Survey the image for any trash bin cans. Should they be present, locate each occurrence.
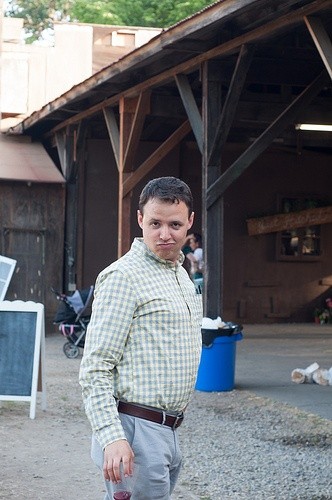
[196,322,243,391]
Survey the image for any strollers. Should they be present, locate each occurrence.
[49,283,95,359]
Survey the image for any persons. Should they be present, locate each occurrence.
[184,234,203,274]
[78,175,203,500]
[179,244,198,278]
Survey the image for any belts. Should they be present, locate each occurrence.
[116,399,184,430]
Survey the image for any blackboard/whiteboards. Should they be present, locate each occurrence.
[0,301,46,401]
